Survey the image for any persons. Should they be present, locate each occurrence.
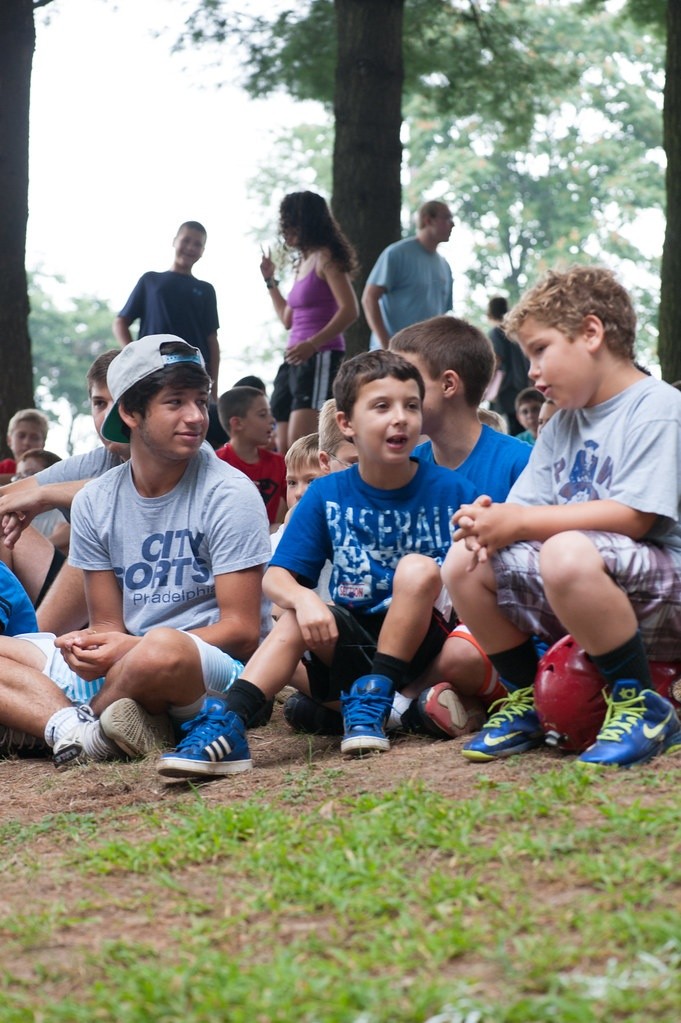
[277,315,552,739]
[0,333,272,771]
[362,199,454,350]
[482,296,537,436]
[0,346,680,724]
[440,266,681,767]
[259,190,361,455]
[156,348,481,774]
[112,220,221,405]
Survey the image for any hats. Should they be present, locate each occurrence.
[100,334,206,444]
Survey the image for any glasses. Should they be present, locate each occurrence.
[11,472,35,482]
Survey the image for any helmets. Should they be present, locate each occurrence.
[535,635,676,754]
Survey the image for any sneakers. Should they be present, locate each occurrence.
[52,697,175,774]
[461,677,544,763]
[282,692,345,736]
[339,674,401,753]
[401,682,486,739]
[574,680,681,772]
[157,697,253,778]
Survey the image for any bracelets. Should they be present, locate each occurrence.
[266,275,281,289]
[307,337,321,352]
